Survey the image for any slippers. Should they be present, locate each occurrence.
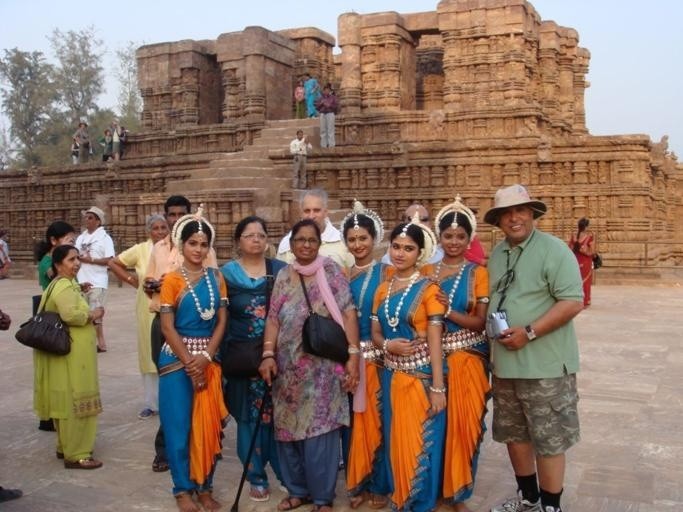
[248,484,270,502]
[96,345,107,352]
[310,504,334,512]
[277,495,312,512]
[369,494,387,509]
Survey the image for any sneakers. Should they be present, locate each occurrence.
[489,489,542,512]
[137,408,154,420]
[151,456,170,472]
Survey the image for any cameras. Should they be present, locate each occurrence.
[486,312,511,338]
[149,280,161,293]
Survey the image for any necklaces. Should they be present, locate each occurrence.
[345,259,376,318]
[178,261,220,322]
[433,256,467,321]
[439,257,469,269]
[181,264,204,276]
[383,266,421,333]
[353,261,374,270]
[390,271,415,283]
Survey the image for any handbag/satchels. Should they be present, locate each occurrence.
[222,339,263,380]
[14,275,73,356]
[301,311,350,365]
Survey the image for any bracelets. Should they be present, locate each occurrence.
[199,349,213,363]
[427,383,445,394]
[523,322,537,343]
[88,255,96,264]
[124,271,135,285]
[258,338,278,366]
[380,336,392,360]
[346,340,359,364]
[443,303,452,320]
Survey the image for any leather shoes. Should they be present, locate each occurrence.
[55,450,95,459]
[63,458,104,470]
[0,485,23,501]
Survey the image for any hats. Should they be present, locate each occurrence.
[483,183,549,227]
[80,206,106,226]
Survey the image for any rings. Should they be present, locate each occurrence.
[193,366,206,390]
[341,369,359,392]
[432,405,441,412]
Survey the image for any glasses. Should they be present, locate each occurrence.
[293,236,320,246]
[240,232,266,241]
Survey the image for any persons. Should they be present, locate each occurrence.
[422,212,489,511]
[220,215,290,502]
[33,219,81,289]
[339,213,394,511]
[258,219,360,511]
[157,221,228,512]
[143,196,215,473]
[106,215,164,420]
[98,130,111,160]
[289,129,311,189]
[72,206,113,355]
[0,309,22,504]
[314,83,338,148]
[119,126,126,159]
[74,119,91,162]
[302,70,318,119]
[274,189,352,266]
[486,187,585,511]
[292,80,303,118]
[37,245,103,469]
[109,117,124,162]
[0,229,11,277]
[568,219,595,306]
[371,221,445,512]
[70,137,80,163]
